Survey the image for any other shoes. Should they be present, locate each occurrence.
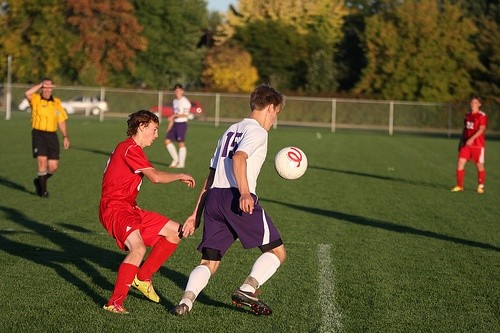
[476,187,484,193]
[34,178,48,198]
[450,185,465,192]
[169,160,185,169]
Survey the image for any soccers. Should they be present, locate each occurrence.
[274,146,308,180]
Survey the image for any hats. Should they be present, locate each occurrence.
[174,84,182,89]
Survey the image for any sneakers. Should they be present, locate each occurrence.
[173,302,189,316]
[231,286,273,316]
[131,273,161,303]
[102,304,130,314]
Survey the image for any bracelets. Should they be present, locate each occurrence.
[63,136,68,138]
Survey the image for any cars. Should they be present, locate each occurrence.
[148,98,204,121]
[18,96,75,115]
[66,95,110,117]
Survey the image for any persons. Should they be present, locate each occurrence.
[173,85,286,316]
[24,78,70,198]
[99,109,196,315]
[165,83,192,169]
[450,96,487,194]
